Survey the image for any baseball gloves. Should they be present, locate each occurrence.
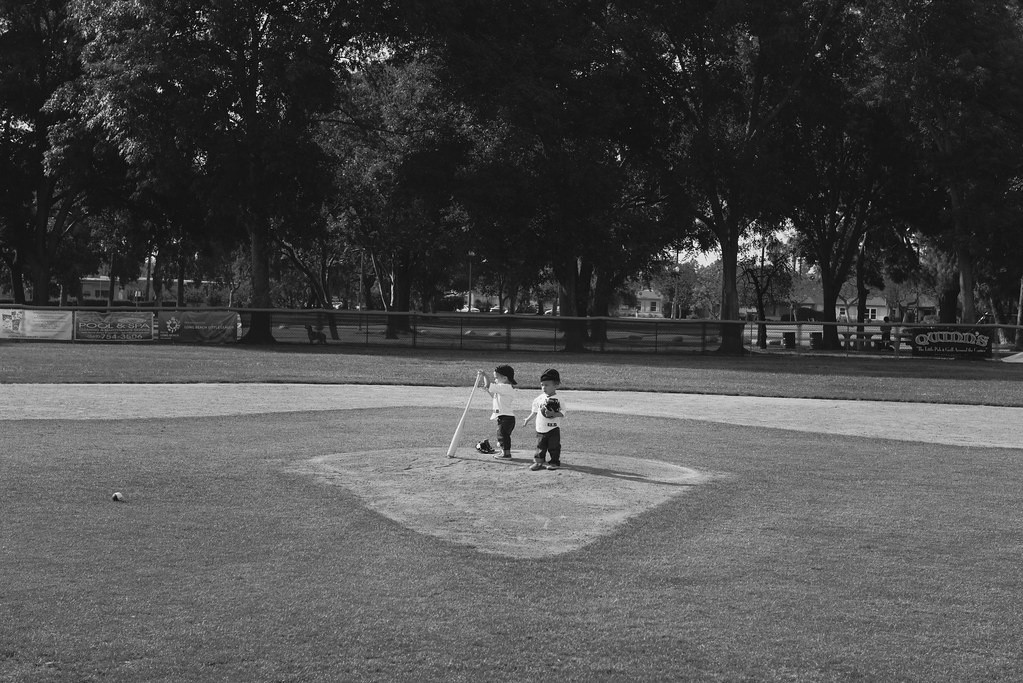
[541,398,561,418]
[475,439,495,454]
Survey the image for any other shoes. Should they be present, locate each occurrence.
[530,462,543,470]
[494,453,511,460]
[547,463,559,470]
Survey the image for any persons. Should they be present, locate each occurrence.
[477,364,517,459]
[522,368,565,471]
[881,318,891,340]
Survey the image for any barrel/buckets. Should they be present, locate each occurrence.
[809,332,822,349]
[782,332,796,351]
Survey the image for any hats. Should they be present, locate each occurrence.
[540,369,562,385]
[494,365,517,385]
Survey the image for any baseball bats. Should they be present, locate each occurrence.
[447,369,483,457]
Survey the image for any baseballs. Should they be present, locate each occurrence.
[112,492,123,501]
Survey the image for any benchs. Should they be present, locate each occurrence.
[840,339,872,349]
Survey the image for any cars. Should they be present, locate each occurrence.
[457,305,481,312]
[490,305,510,313]
[545,305,560,316]
